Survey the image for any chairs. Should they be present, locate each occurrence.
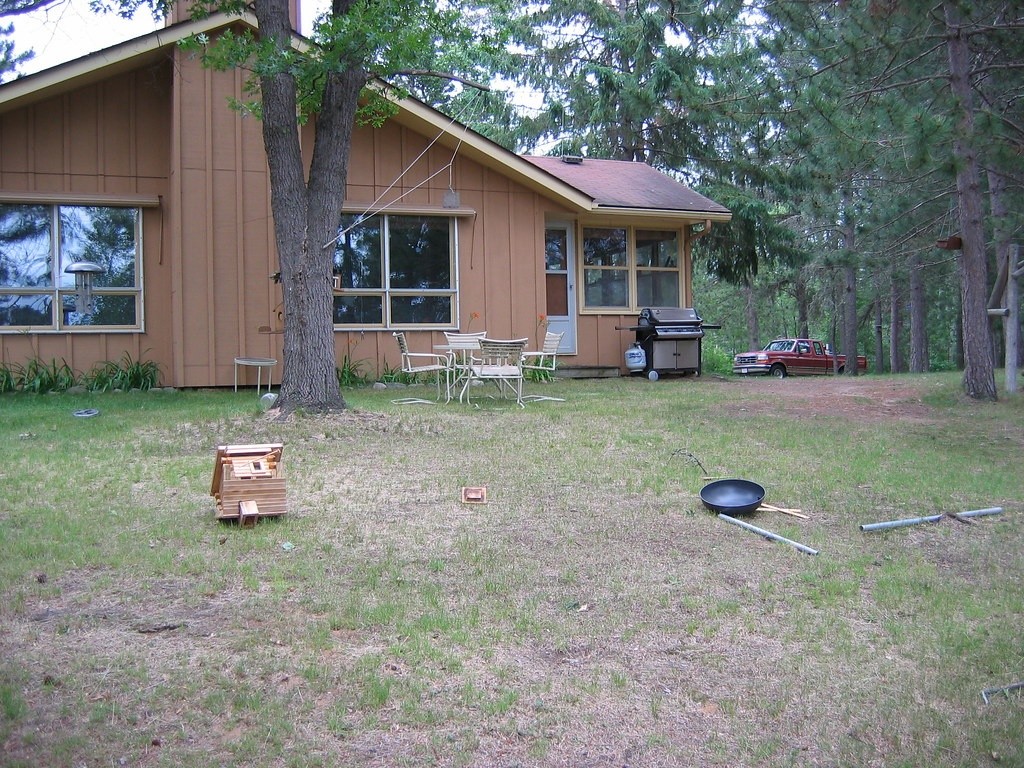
[391,330,566,411]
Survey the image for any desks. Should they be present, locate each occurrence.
[433,343,529,404]
[235,357,281,396]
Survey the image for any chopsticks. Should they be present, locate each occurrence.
[754,503,810,520]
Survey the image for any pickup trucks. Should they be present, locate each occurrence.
[731,338,867,379]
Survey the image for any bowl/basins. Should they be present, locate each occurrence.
[699,478,766,515]
